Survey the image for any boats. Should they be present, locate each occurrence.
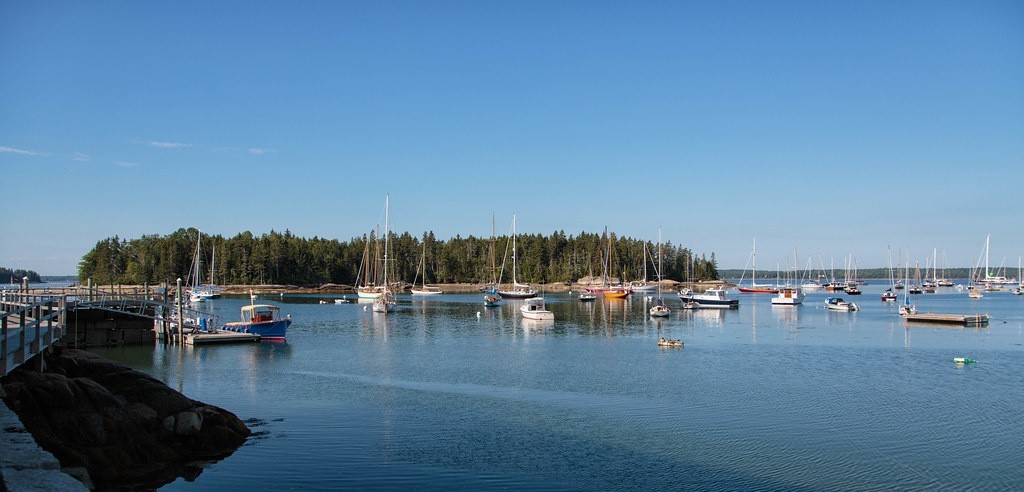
[678,286,740,309]
[173,290,192,310]
[520,276,554,319]
[683,302,699,309]
[657,339,685,346]
[772,287,806,305]
[824,296,852,310]
[222,288,292,343]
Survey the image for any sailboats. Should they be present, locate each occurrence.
[410,242,445,296]
[478,243,502,294]
[497,212,539,299]
[778,233,1024,302]
[484,212,505,307]
[352,223,414,302]
[649,227,671,317]
[577,231,683,301]
[181,229,207,303]
[194,245,222,299]
[372,193,398,314]
[897,250,918,315]
[736,238,779,293]
